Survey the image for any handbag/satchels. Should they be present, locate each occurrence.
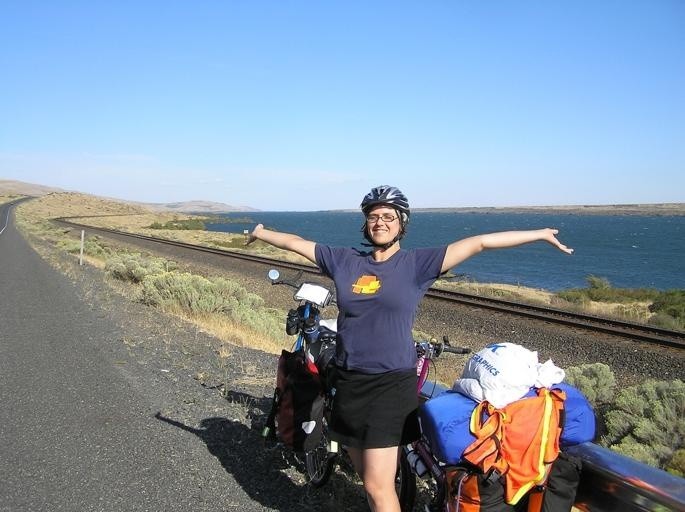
[453,340,567,414]
[275,346,325,454]
[442,449,581,512]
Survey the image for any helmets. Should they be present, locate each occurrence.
[362,184,411,217]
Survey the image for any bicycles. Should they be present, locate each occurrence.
[394,333,474,512]
[266,271,345,488]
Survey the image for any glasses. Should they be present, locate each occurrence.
[363,212,399,223]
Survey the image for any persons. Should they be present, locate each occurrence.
[244,186,575,512]
